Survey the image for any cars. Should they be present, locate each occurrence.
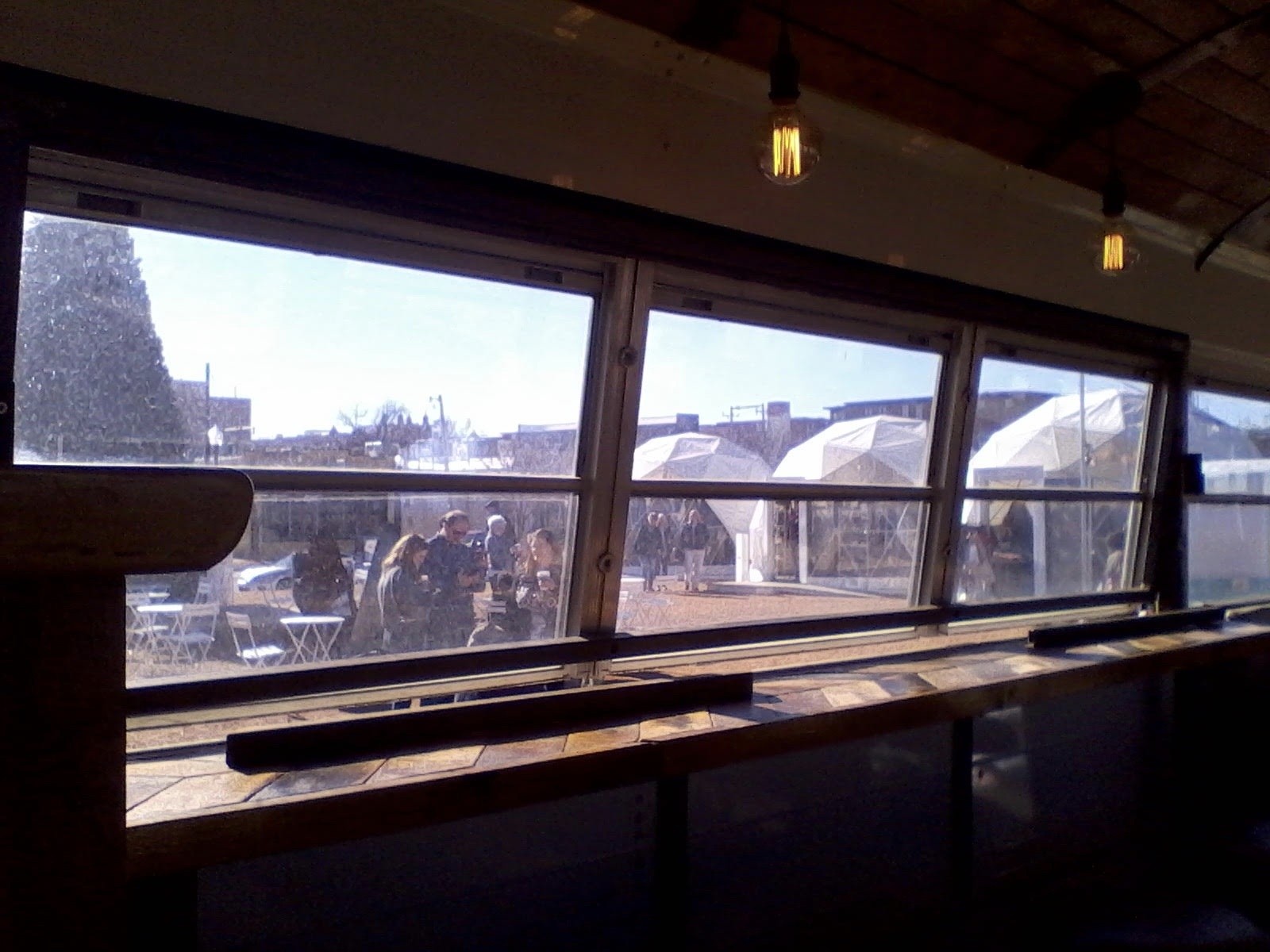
[237,552,355,591]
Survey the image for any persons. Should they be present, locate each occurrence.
[292,498,1127,712]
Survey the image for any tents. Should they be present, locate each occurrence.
[631,388,1269,620]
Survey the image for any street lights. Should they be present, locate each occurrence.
[206,424,224,465]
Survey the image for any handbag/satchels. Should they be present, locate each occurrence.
[972,558,996,585]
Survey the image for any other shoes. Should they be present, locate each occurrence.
[648,586,654,591]
[685,584,689,590]
[692,588,699,593]
[643,581,647,591]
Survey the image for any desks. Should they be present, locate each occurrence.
[279,616,345,662]
[135,604,186,664]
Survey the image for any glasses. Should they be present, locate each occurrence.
[448,525,466,537]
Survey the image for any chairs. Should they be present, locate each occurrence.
[123,583,286,669]
